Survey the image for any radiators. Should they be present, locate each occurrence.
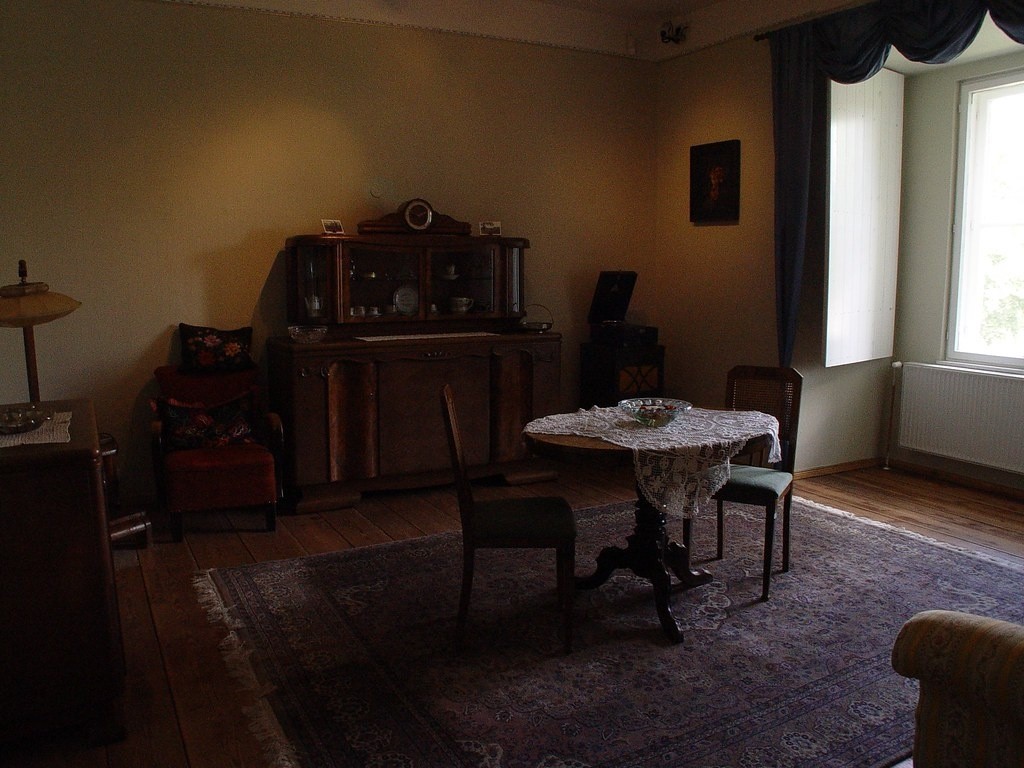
[882,361,1024,476]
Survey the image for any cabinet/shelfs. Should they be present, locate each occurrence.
[0,398,127,748]
[581,342,664,408]
[266,234,564,518]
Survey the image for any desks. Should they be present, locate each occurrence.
[526,408,770,643]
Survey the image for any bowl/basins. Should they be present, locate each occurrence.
[618,398,692,426]
[287,325,328,342]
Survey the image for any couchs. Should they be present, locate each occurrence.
[890,610,1024,768]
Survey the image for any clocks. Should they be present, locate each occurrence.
[357,198,472,236]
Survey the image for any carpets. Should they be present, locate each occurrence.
[191,499,1024,768]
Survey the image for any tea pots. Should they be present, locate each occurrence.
[305,294,324,316]
[450,298,474,311]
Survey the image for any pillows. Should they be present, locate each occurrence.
[178,322,260,373]
[148,386,260,452]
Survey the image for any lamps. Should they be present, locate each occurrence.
[0,258,82,402]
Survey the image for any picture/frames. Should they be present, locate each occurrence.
[689,139,740,227]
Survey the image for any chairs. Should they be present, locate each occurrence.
[440,381,578,655]
[146,366,288,542]
[683,365,805,602]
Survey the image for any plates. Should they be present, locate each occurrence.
[353,314,366,317]
[393,285,421,316]
[366,314,382,317]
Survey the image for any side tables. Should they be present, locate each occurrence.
[97,433,151,545]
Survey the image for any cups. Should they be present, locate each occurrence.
[354,305,365,314]
[369,307,380,314]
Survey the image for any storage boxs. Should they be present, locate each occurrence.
[588,271,659,341]
